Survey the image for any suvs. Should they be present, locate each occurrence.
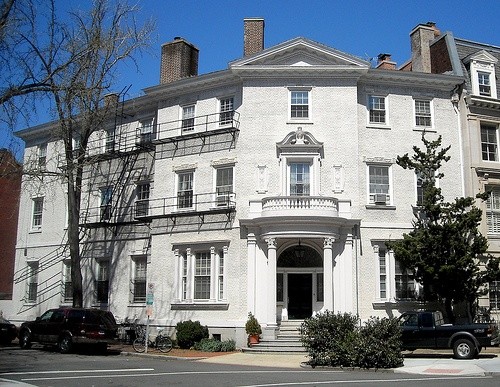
[17,308,120,356]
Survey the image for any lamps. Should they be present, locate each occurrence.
[294,238,305,259]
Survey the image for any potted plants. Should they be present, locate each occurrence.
[245,311,262,344]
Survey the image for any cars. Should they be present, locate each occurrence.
[0,316,18,344]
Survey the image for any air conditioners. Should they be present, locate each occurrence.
[217,196,229,205]
[374,194,386,202]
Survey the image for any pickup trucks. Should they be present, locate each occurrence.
[385,311,497,360]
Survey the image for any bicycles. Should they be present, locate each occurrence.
[132,327,173,353]
